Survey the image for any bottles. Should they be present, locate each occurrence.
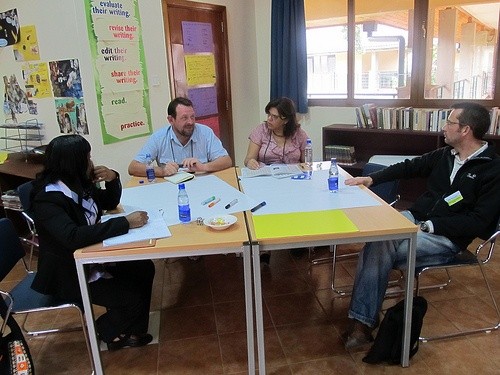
[177,183,191,223]
[304,139,312,168]
[145,154,156,183]
[329,158,338,192]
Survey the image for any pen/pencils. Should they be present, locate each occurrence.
[179,163,196,168]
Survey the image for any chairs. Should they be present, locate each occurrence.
[384,212,500,343]
[306,162,405,297]
[0,217,97,375]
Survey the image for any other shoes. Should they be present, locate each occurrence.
[341,313,380,350]
[259,251,270,265]
[95,322,152,351]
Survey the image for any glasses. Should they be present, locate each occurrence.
[266,112,281,120]
[445,118,466,126]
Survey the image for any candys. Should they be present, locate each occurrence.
[210,218,229,226]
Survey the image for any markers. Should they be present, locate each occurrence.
[251,202,266,212]
[225,199,237,209]
[202,196,215,205]
[209,198,221,207]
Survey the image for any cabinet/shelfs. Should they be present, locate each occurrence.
[322,124,500,210]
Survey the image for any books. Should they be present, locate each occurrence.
[359,104,453,132]
[164,171,195,184]
[325,145,356,166]
[485,107,500,135]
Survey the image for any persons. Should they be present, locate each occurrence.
[245,97,308,264]
[128,97,233,176]
[342,101,500,349]
[30,134,155,349]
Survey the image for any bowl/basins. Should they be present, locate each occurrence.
[203,215,238,230]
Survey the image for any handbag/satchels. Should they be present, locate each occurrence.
[0,332,34,375]
[361,296,428,365]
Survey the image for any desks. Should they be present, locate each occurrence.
[0,151,44,235]
[73,166,256,375]
[235,161,419,375]
